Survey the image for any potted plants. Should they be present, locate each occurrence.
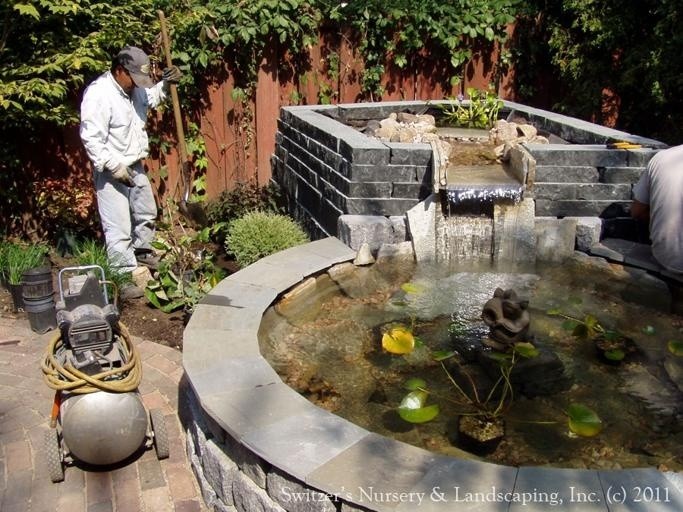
[7,244,46,312]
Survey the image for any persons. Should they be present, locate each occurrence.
[79,45,182,301]
[630,143,683,274]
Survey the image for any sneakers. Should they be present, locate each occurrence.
[118,281,143,300]
[134,250,160,269]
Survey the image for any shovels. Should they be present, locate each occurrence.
[156,9,209,231]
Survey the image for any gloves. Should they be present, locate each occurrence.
[162,65,180,82]
[111,162,136,187]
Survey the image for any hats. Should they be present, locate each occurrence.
[117,46,153,88]
